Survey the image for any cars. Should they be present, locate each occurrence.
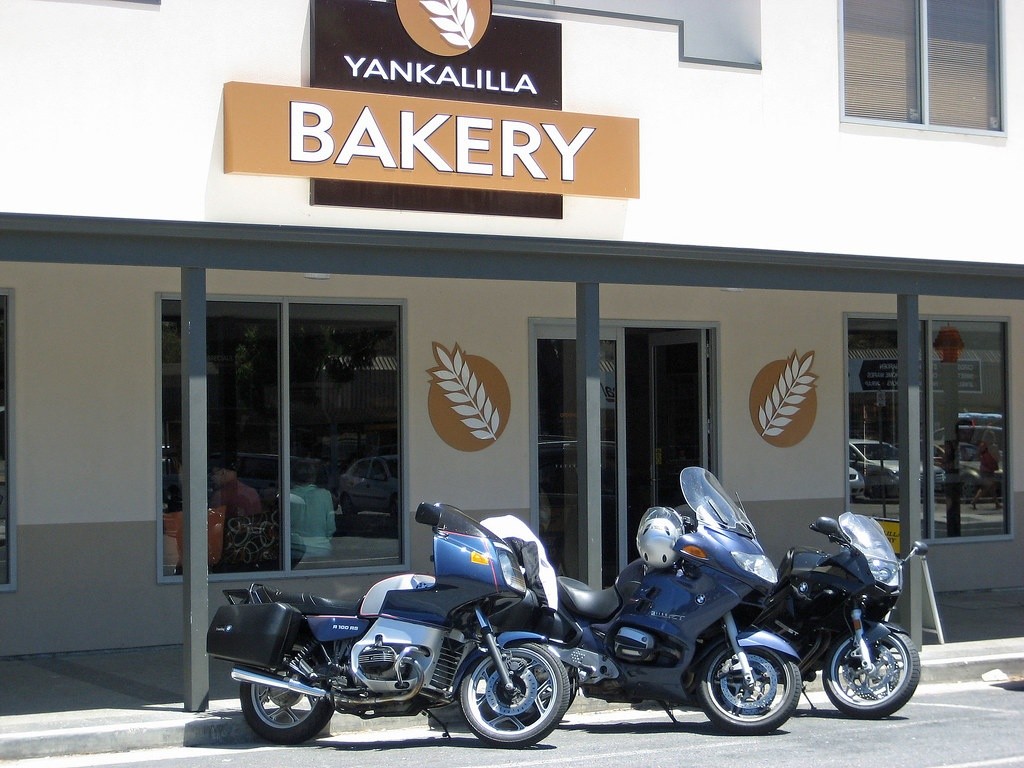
[848,464,864,503]
[161,455,182,513]
[335,452,399,523]
[848,435,947,500]
[889,436,1005,498]
[538,433,618,547]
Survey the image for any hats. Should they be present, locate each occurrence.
[212,455,237,470]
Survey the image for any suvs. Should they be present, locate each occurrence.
[211,444,341,512]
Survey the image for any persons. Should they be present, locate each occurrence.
[290,463,337,561]
[972,429,1003,510]
[207,453,262,520]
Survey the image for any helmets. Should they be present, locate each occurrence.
[636,506,684,569]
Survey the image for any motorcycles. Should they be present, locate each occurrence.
[697,511,932,720]
[203,500,573,750]
[477,464,804,735]
